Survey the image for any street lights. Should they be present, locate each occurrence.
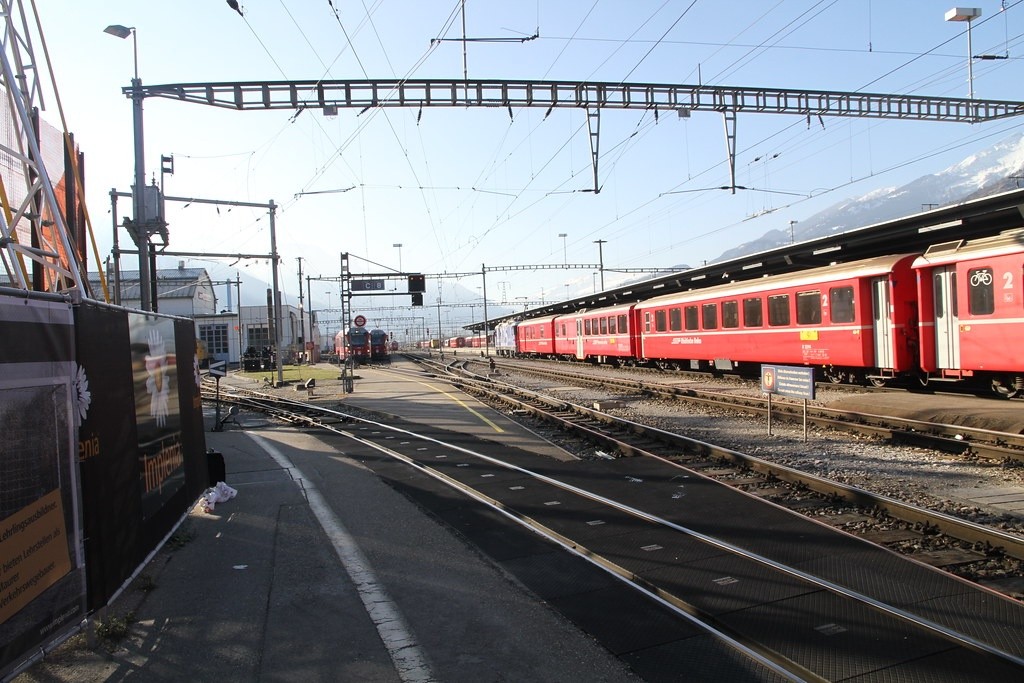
[101,25,168,313]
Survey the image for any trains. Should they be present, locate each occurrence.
[368,329,391,364]
[332,327,371,364]
[494,223,1024,403]
[386,332,495,352]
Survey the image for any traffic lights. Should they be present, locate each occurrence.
[405,329,408,335]
[390,331,392,339]
[426,328,429,335]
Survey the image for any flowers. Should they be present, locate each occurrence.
[50,361,92,429]
[193,353,200,388]
[144,329,171,429]
